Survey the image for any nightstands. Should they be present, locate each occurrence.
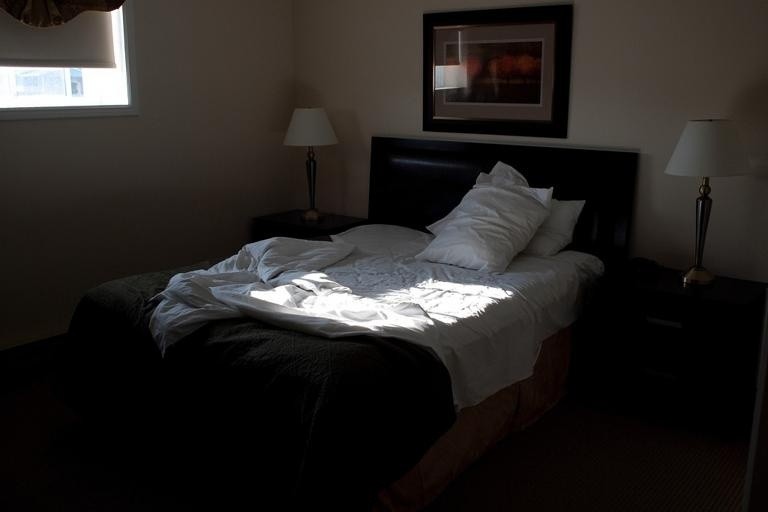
[243,209,365,251]
[619,257,767,445]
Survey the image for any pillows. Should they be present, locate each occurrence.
[414,172,554,275]
[426,186,586,257]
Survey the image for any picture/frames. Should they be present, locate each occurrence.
[422,3,573,139]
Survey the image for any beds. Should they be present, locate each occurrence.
[48,136,638,512]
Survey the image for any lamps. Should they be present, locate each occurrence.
[664,119,750,287]
[282,108,339,221]
